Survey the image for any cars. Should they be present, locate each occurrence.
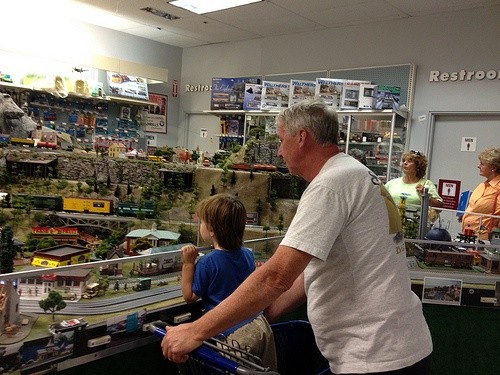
[48,317,89,336]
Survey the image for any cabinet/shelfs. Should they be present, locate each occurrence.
[185,110,405,183]
[0,82,150,159]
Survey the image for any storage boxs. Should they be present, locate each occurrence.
[412,245,476,269]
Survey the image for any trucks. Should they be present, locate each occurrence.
[133,278,152,292]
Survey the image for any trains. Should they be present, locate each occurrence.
[0,192,155,218]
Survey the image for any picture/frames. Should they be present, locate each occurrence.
[144,91,168,134]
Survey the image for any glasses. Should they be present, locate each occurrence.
[410,150,420,155]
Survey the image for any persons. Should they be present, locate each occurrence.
[460,144,500,242]
[384,149,444,252]
[181,193,276,372]
[348,148,368,166]
[161,98,437,375]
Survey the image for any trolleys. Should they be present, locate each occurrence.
[142,319,332,375]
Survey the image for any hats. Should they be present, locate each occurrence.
[426,228,451,241]
